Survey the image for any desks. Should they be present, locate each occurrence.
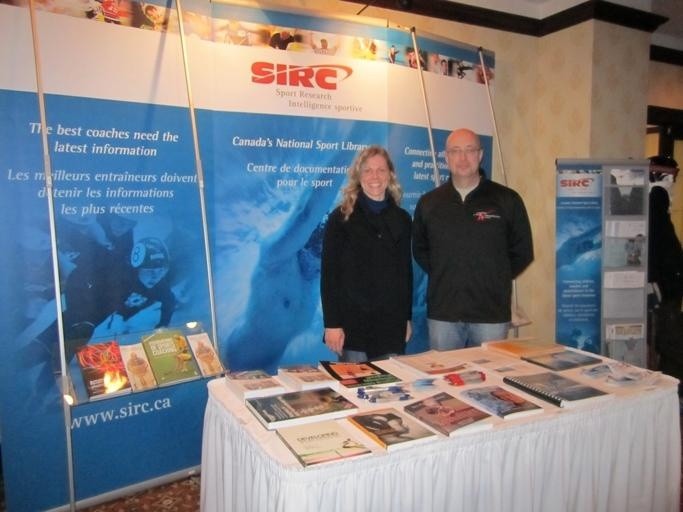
[199,338,682,512]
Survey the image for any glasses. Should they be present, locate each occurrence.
[445,147,480,156]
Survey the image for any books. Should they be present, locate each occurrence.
[221,332,660,464]
[69,329,223,402]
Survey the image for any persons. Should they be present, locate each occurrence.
[322,142,415,365]
[560,327,601,355]
[623,236,644,265]
[83,0,495,86]
[223,119,374,376]
[556,224,604,268]
[411,127,537,351]
